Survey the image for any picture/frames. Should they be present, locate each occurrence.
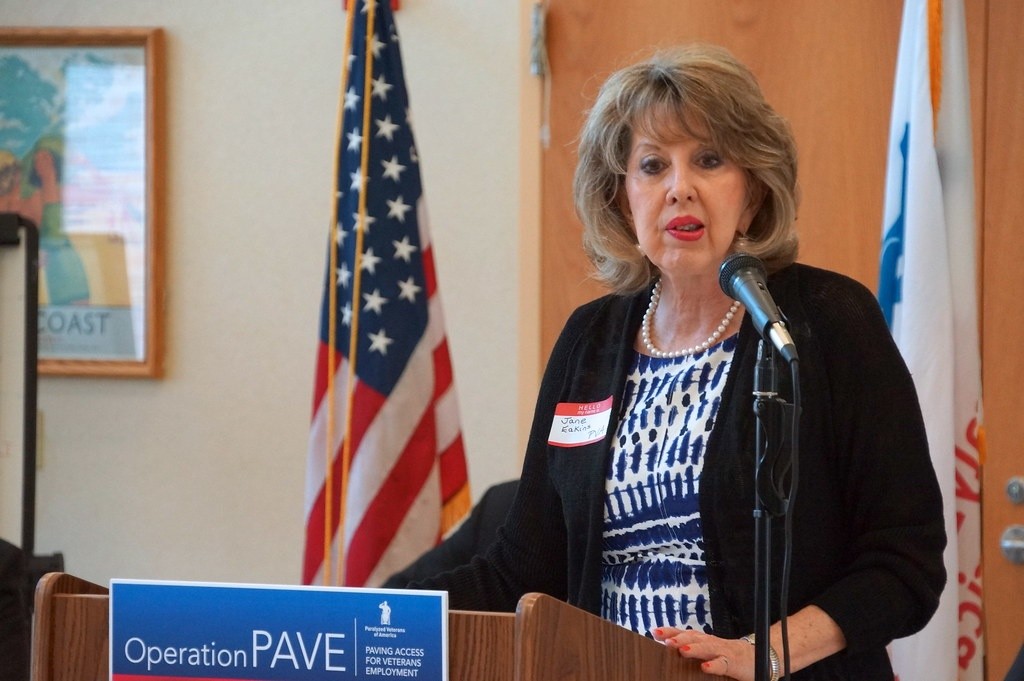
[0,25,168,381]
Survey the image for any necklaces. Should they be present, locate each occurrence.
[642,278,741,358]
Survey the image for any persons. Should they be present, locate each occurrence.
[378,42,949,681]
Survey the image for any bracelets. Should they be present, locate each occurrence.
[740,634,780,681]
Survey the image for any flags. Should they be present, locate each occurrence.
[876,1,983,681]
[300,1,472,588]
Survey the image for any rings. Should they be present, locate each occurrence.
[719,656,729,674]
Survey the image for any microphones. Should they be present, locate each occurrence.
[718,250,800,368]
